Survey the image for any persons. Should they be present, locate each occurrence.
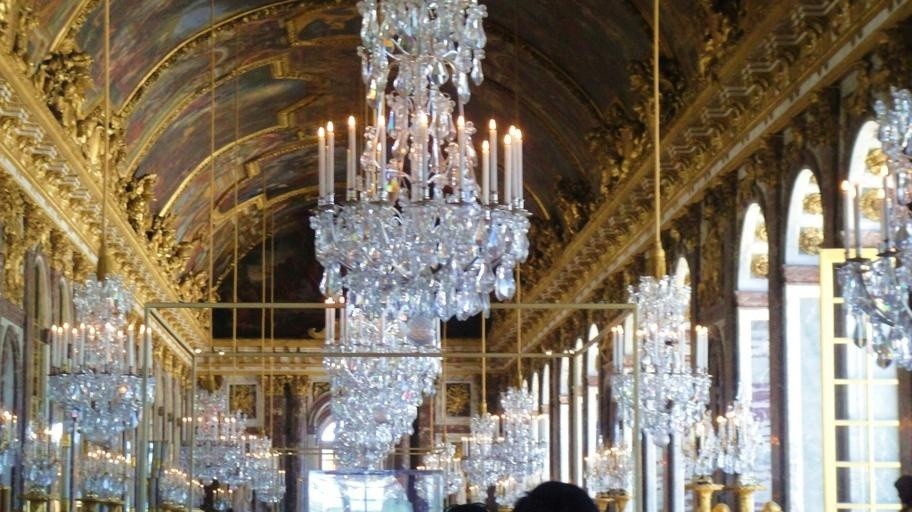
[443,502,491,512]
[512,480,600,512]
[894,474,912,512]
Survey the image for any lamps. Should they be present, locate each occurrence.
[189,2,289,506]
[608,3,715,447]
[44,2,156,445]
[306,1,536,325]
